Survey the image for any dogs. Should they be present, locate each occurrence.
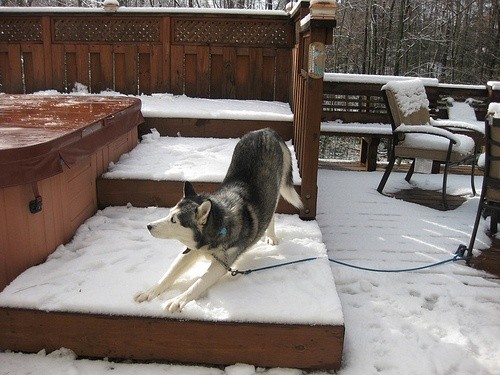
[134,126,305,313]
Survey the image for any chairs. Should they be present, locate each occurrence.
[376,79,484,208]
[466,111,500,265]
